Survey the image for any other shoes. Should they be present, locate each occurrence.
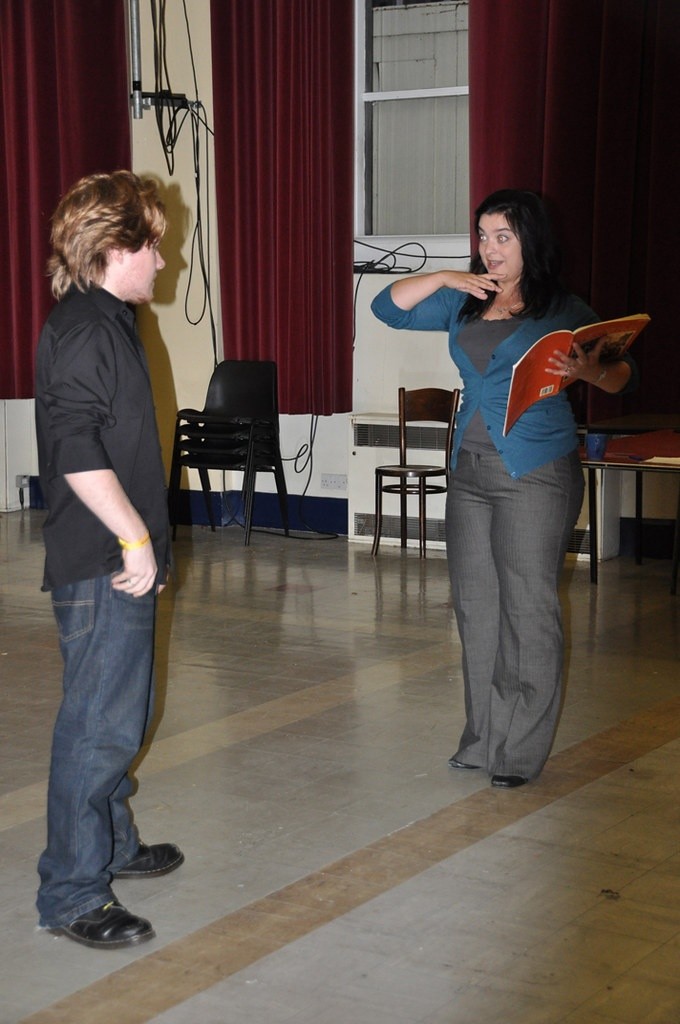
[448,758,481,769]
[491,774,529,788]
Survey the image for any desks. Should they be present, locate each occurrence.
[577,455,680,596]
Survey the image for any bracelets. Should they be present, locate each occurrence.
[115,530,149,549]
[591,365,608,385]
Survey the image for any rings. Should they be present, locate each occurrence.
[128,579,133,586]
[565,366,568,371]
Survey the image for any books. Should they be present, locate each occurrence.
[502,314,650,438]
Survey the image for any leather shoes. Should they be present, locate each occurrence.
[113,840,184,879]
[46,899,155,949]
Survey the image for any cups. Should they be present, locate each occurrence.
[585,433,608,461]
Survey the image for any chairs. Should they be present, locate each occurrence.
[371,387,460,556]
[169,360,289,549]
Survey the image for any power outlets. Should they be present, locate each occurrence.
[320,473,349,489]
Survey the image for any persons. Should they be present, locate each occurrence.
[370,186,637,789]
[32,169,186,951]
